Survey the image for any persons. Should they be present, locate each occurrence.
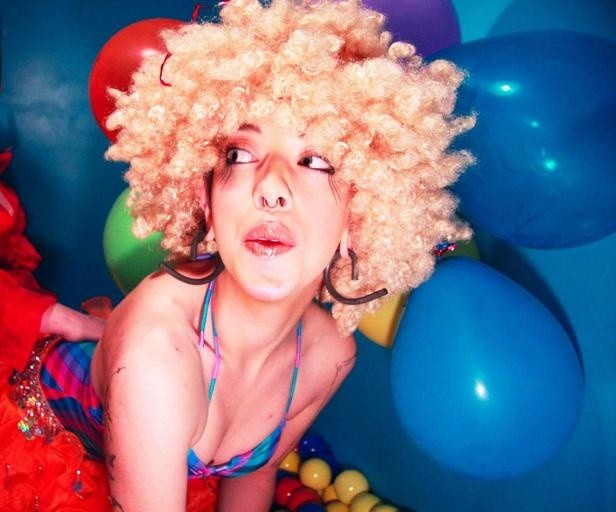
[0,0,479,509]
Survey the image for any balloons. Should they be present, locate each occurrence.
[391,254,582,480]
[358,285,407,349]
[103,183,174,299]
[294,0,462,59]
[419,29,615,249]
[88,16,192,148]
